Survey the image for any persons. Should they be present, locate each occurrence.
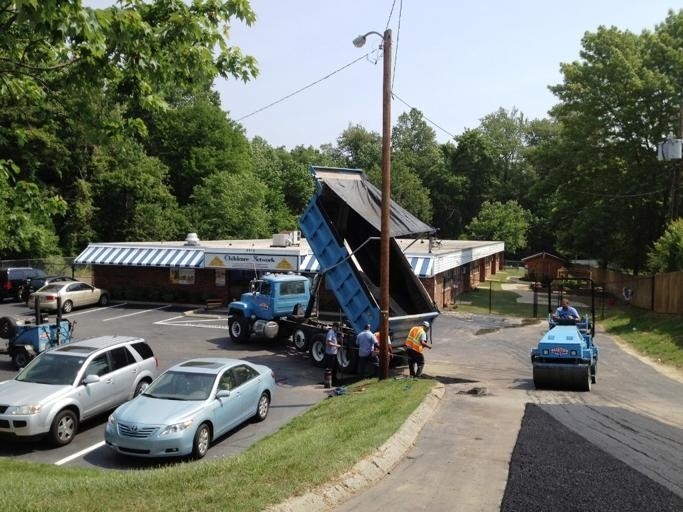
[324,323,341,386]
[355,323,379,379]
[373,326,393,363]
[403,321,431,377]
[552,298,582,322]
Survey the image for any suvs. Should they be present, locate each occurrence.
[0,334,159,448]
[20,275,78,306]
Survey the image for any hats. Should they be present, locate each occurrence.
[424,321,429,327]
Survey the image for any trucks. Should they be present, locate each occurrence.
[226,163,443,379]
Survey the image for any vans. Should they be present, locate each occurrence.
[0,267,47,302]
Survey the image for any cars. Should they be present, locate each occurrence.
[26,280,111,314]
[104,355,277,462]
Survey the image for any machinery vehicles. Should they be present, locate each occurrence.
[0,314,72,366]
[527,312,599,392]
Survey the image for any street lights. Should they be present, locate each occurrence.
[350,28,395,382]
